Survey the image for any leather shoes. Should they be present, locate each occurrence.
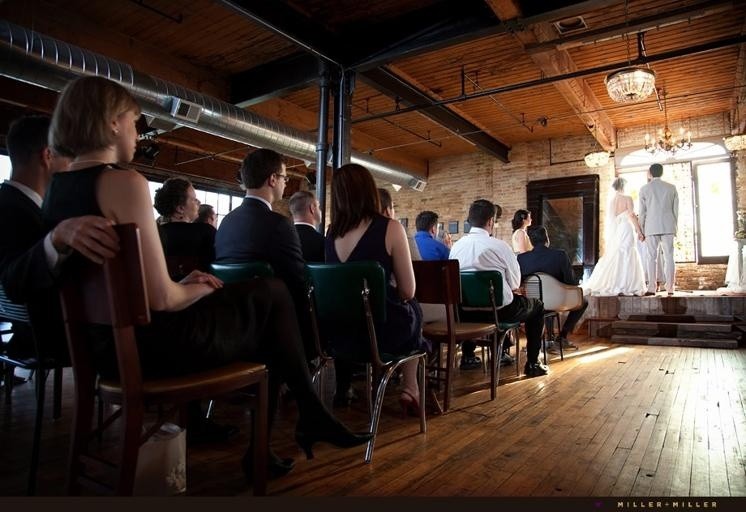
[644,291,656,296]
[524,362,546,377]
[499,353,517,368]
[333,393,362,410]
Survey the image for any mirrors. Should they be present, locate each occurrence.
[526,174,600,284]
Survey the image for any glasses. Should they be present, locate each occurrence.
[276,173,290,182]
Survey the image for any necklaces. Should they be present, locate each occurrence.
[68,160,108,166]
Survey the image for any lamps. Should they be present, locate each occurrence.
[643,93,694,154]
[584,119,609,169]
[602,10,656,105]
[721,108,746,154]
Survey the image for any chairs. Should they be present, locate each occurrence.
[62,223,268,502]
[0,293,105,500]
[526,270,584,356]
[305,264,428,461]
[519,273,564,365]
[408,259,500,412]
[454,269,522,386]
[207,262,324,456]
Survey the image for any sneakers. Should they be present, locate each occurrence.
[547,334,576,352]
[460,356,483,370]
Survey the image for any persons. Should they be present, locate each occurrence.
[578,178,647,297]
[639,170,667,292]
[39,75,374,480]
[153,148,588,418]
[638,164,679,296]
[0,115,240,445]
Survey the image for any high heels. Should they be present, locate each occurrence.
[241,457,296,487]
[398,390,427,420]
[293,421,375,461]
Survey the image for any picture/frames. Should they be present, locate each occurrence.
[463,220,472,232]
[438,222,444,239]
[448,221,458,234]
[399,217,408,228]
